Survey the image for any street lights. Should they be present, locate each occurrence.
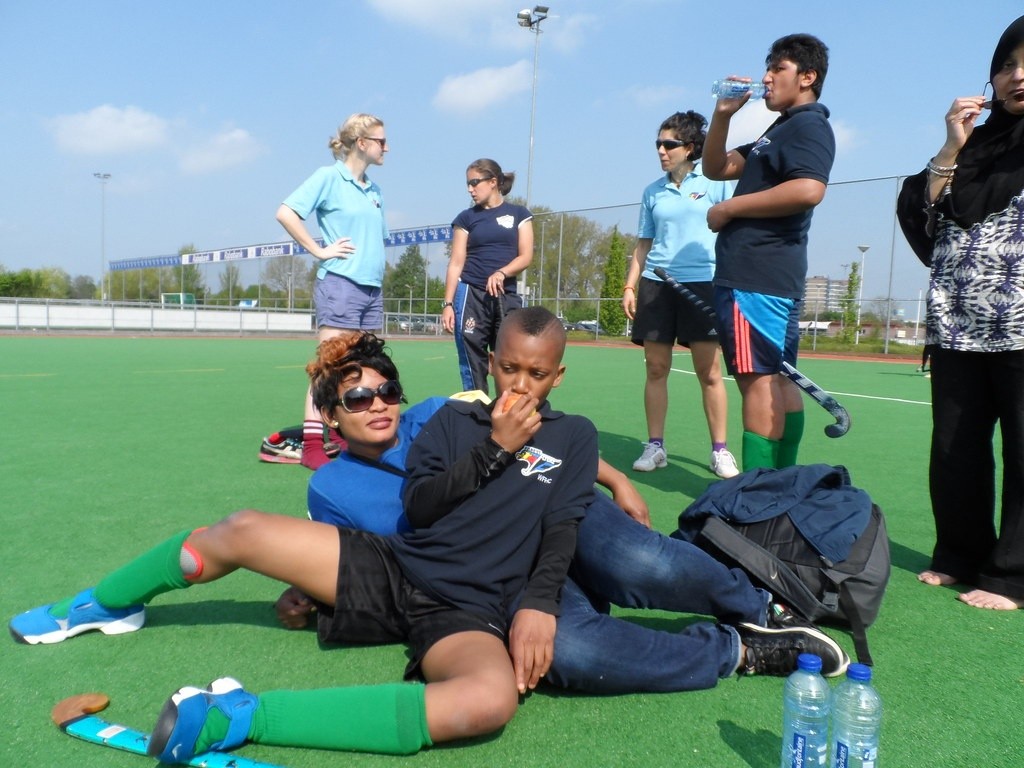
[539,219,551,306]
[405,284,412,335]
[93,172,110,307]
[517,4,550,304]
[856,245,871,344]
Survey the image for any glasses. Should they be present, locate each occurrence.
[981,81,1024,110]
[467,177,492,187]
[356,137,386,148]
[336,381,400,412]
[655,139,686,150]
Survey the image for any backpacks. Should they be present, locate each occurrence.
[668,501,891,627]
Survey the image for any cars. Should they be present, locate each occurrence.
[559,317,606,335]
[386,316,435,330]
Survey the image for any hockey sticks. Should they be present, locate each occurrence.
[497,289,505,319]
[52,693,288,768]
[653,265,850,438]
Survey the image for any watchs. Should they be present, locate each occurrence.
[441,301,452,308]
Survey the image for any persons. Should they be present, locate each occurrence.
[276,334,849,693]
[898,14,1024,611]
[275,114,388,471]
[441,160,533,397]
[7,304,597,761]
[620,110,739,480]
[703,33,836,472]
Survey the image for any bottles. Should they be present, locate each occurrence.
[830,663,883,768]
[710,79,768,101]
[779,653,830,768]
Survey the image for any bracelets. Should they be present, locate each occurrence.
[495,270,506,279]
[926,164,950,177]
[927,156,957,171]
[623,286,635,293]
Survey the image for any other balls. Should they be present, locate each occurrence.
[503,394,536,418]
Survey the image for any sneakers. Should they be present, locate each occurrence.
[709,447,739,477]
[259,437,341,463]
[731,601,850,678]
[147,676,260,763]
[9,588,146,644]
[632,441,668,472]
